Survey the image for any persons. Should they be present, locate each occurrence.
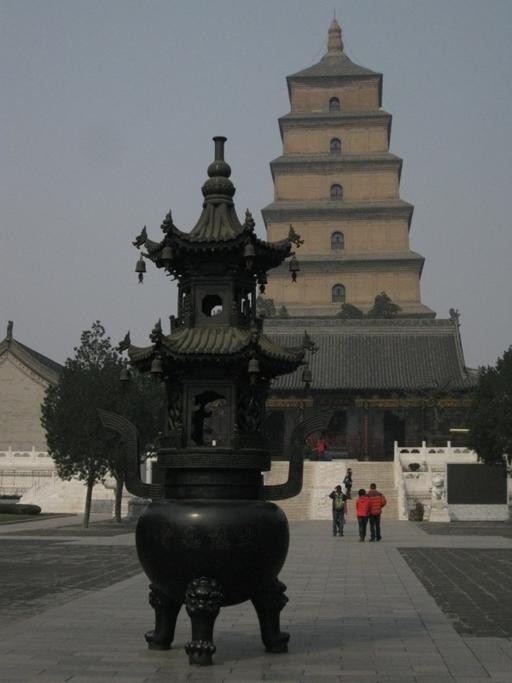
[329,485,347,536]
[343,468,352,499]
[355,483,386,542]
